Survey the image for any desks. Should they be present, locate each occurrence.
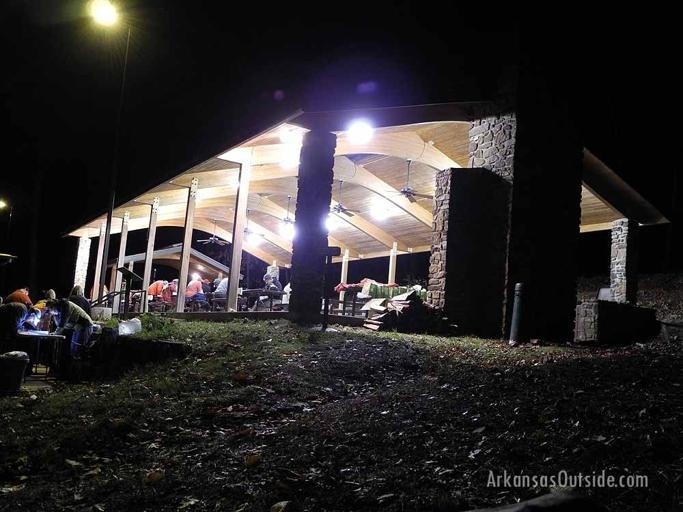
[339,283,398,317]
[18,329,66,383]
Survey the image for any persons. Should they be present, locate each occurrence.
[34,289,56,308]
[148,270,247,312]
[44,298,94,381]
[247,273,282,311]
[68,285,92,318]
[282,281,292,305]
[0,301,41,354]
[3,286,33,309]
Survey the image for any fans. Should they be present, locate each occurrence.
[329,180,360,224]
[196,210,264,247]
[388,159,432,203]
